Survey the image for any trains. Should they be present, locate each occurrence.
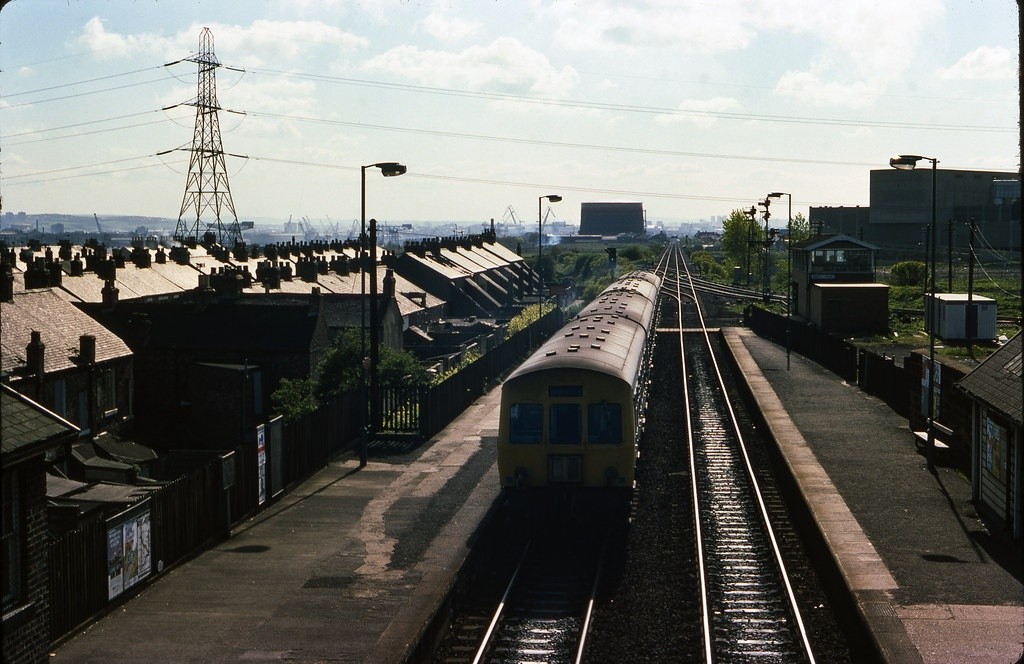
[498,270,662,515]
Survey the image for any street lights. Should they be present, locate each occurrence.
[539,195,562,344]
[890,154,936,468]
[768,193,791,370]
[359,163,407,465]
